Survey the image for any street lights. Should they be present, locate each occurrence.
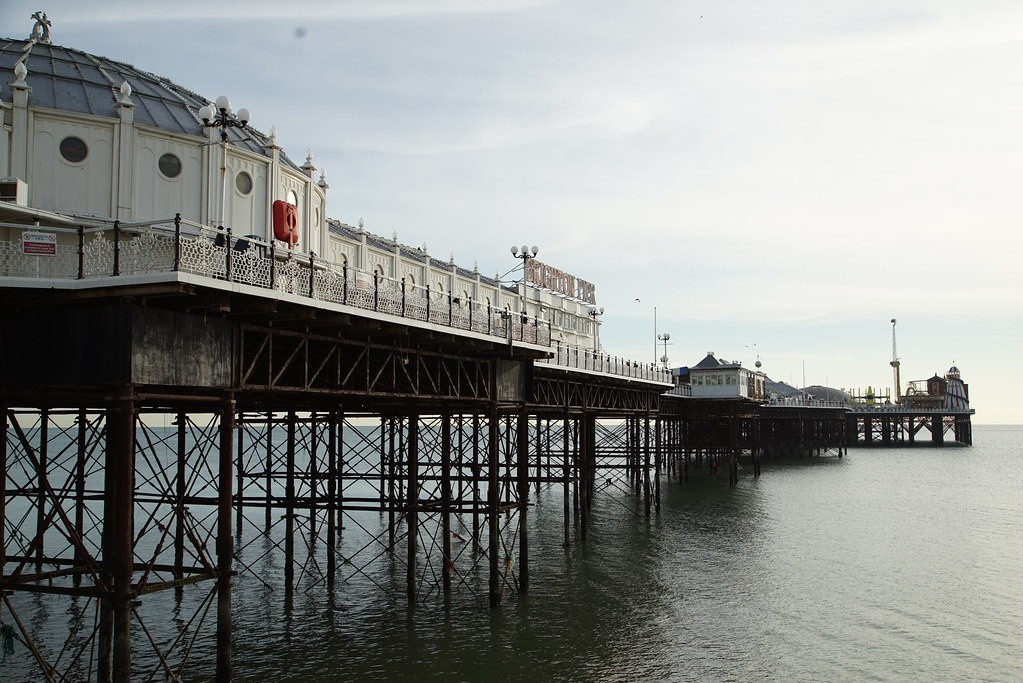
[587,307,604,372]
[510,244,540,324]
[200,96,251,281]
[658,333,670,372]
